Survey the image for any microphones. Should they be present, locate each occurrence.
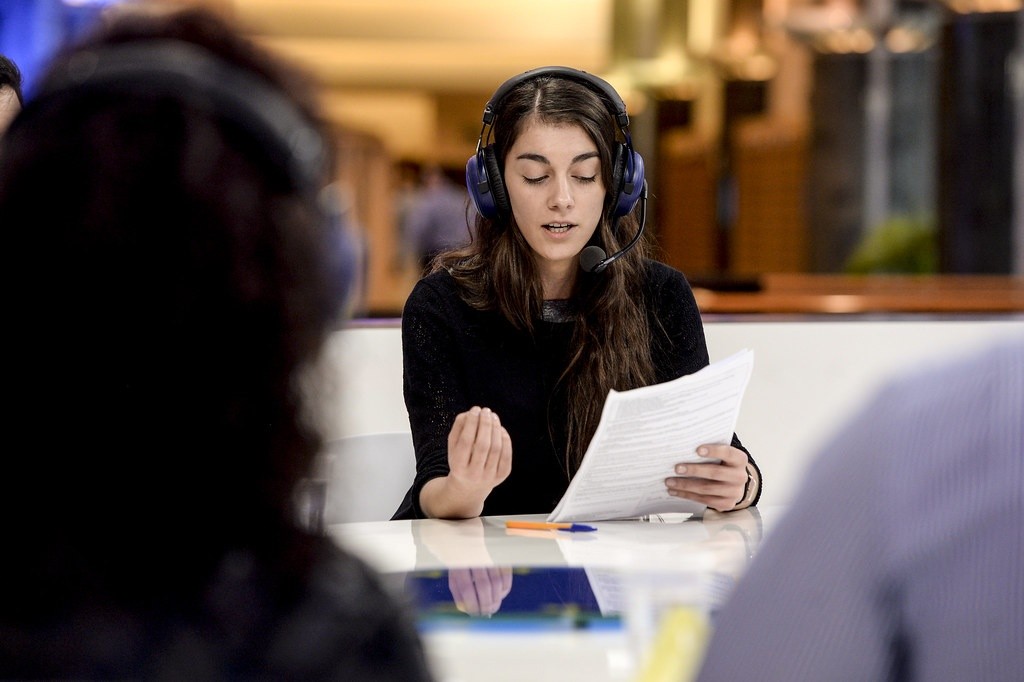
[579,198,648,276]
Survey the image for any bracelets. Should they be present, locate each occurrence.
[732,467,753,506]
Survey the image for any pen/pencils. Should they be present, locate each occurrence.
[506,521,599,534]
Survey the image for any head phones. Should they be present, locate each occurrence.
[464,64,646,221]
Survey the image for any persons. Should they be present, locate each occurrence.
[364,150,478,270]
[687,342,1024,679]
[0,8,437,682]
[0,54,24,132]
[388,67,763,522]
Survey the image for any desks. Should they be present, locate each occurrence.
[318,513,784,682]
